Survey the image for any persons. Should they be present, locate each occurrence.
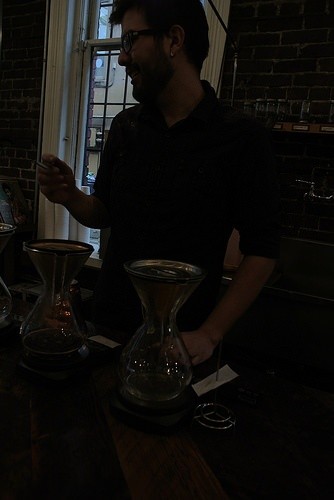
[33,1,288,367]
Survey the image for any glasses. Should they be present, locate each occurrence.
[121,26,164,56]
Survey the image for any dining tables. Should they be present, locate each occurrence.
[0,320,233,500]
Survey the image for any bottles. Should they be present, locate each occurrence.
[22,239,94,355]
[117,258,208,402]
[0,222,17,318]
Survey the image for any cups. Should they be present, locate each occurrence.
[243,97,311,123]
